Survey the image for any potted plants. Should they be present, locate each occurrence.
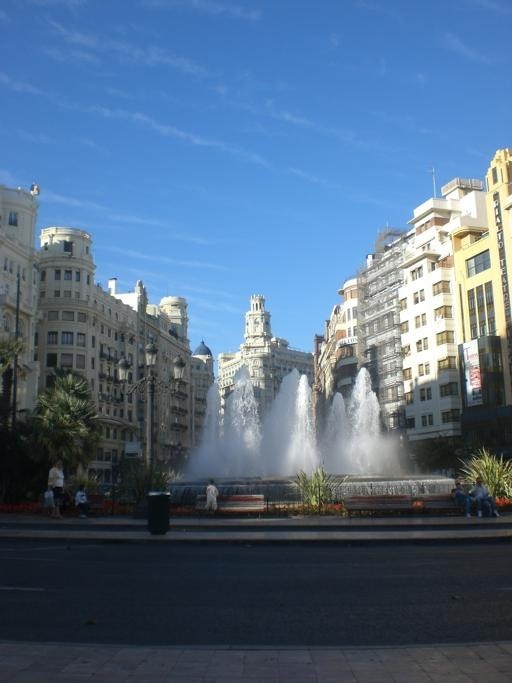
[145,468,172,534]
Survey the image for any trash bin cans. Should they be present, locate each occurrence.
[146,491,172,535]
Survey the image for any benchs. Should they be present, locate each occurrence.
[343,492,410,515]
[422,487,488,515]
[61,494,108,515]
[194,493,267,519]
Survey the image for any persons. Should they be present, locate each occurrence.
[468,475,500,519]
[75,483,90,521]
[47,458,67,520]
[204,478,221,512]
[449,477,477,517]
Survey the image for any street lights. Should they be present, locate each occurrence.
[115,341,187,471]
